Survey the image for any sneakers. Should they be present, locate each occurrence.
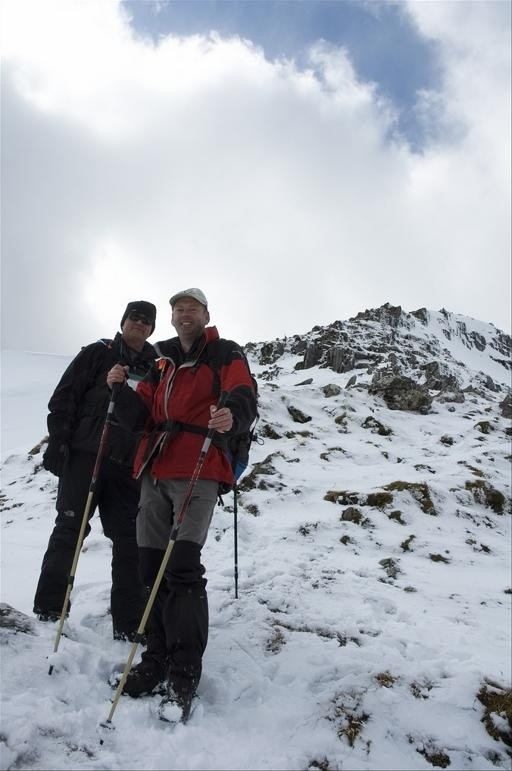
[108,661,198,724]
[37,614,69,622]
[112,627,147,647]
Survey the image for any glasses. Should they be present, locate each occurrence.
[127,313,153,325]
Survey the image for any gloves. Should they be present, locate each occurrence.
[43,435,69,477]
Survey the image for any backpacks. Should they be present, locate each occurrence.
[211,338,258,496]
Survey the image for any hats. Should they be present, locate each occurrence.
[169,288,208,306]
[120,301,156,336]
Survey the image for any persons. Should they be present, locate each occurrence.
[32,301,159,642]
[108,286,257,725]
